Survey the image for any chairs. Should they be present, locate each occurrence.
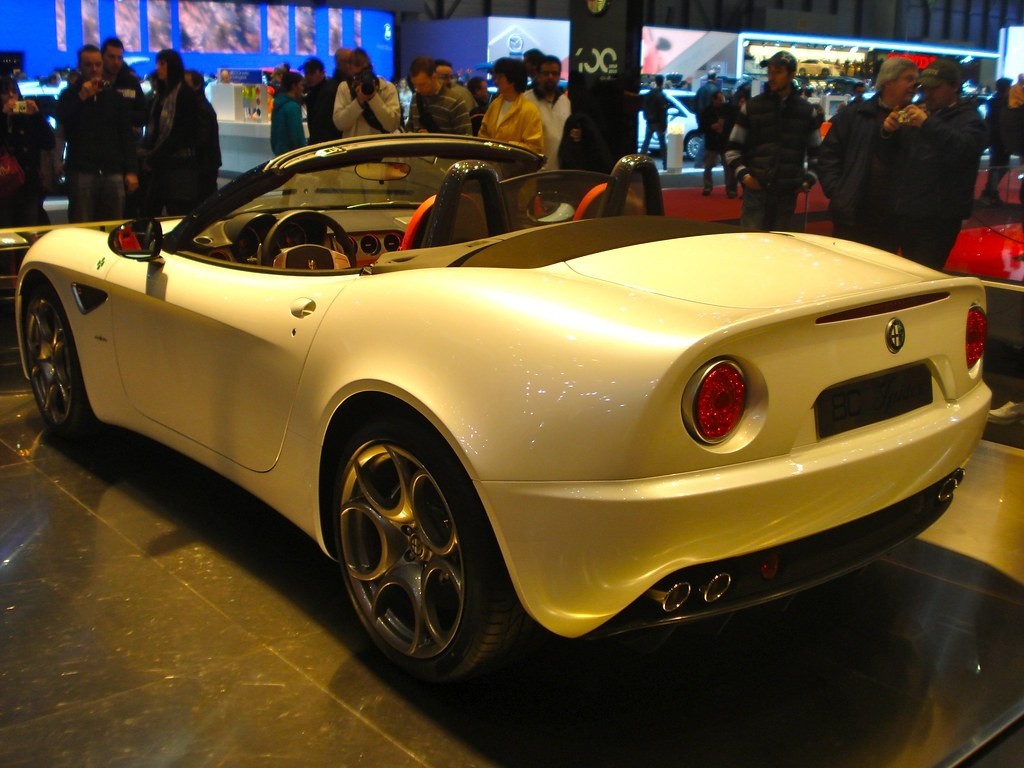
[572,154,664,221]
[400,157,507,250]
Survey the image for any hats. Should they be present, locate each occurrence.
[915,59,963,88]
[760,52,796,73]
[298,57,325,72]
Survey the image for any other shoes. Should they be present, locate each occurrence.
[702,187,712,194]
[987,400,1024,424]
[727,190,737,197]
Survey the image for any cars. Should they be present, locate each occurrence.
[636,86,706,160]
[796,58,838,76]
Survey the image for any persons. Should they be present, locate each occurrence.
[640,75,668,170]
[268,44,582,173]
[678,50,1024,270]
[0,39,234,229]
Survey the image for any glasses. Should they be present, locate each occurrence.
[440,74,453,80]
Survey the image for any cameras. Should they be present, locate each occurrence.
[351,67,379,94]
[897,111,911,123]
[13,102,28,112]
[97,80,112,90]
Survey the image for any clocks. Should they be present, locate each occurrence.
[586,0,610,17]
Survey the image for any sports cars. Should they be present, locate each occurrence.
[14,132,996,697]
